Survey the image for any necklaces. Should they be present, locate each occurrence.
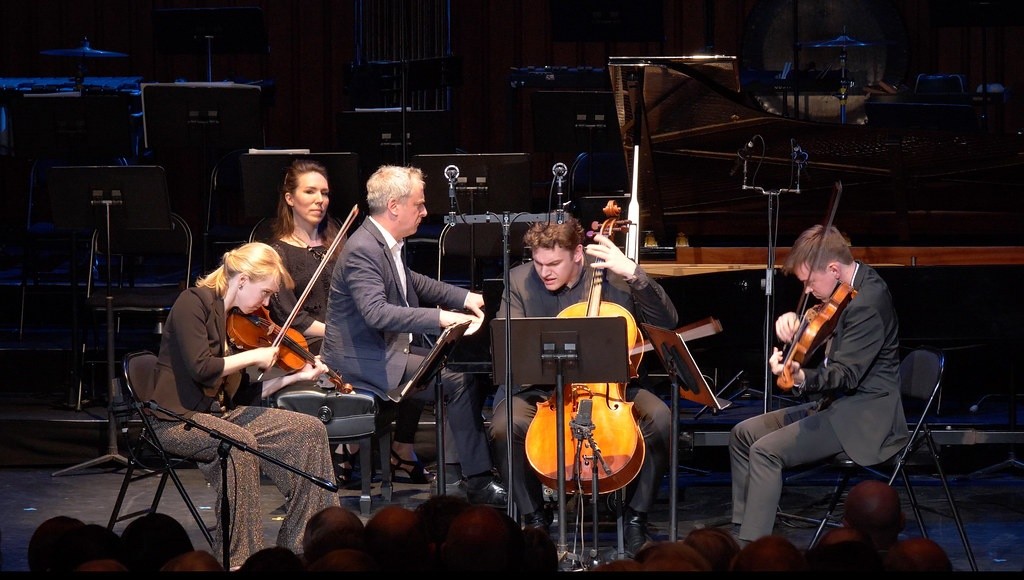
[290,232,327,258]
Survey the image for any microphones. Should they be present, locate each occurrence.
[109,378,130,444]
[791,140,808,169]
[728,134,758,176]
[552,163,568,177]
[574,399,593,450]
[443,165,460,181]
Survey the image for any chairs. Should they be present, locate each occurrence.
[19,39,979,573]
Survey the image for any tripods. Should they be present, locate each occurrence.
[47,165,174,477]
[696,157,846,534]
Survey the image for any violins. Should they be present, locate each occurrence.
[776,280,857,392]
[227,305,353,395]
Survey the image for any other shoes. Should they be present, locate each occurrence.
[728,525,750,549]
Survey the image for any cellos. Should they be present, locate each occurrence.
[524,200,648,569]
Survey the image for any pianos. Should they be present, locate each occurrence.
[608,56,1024,399]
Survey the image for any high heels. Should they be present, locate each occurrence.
[330,444,359,485]
[389,447,436,484]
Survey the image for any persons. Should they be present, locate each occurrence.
[728,225,910,550]
[269,160,437,483]
[143,242,340,571]
[27,483,956,580]
[321,165,509,503]
[846,57,897,125]
[489,211,679,558]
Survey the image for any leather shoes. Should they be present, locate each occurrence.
[623,512,646,558]
[466,480,507,505]
[525,507,548,533]
